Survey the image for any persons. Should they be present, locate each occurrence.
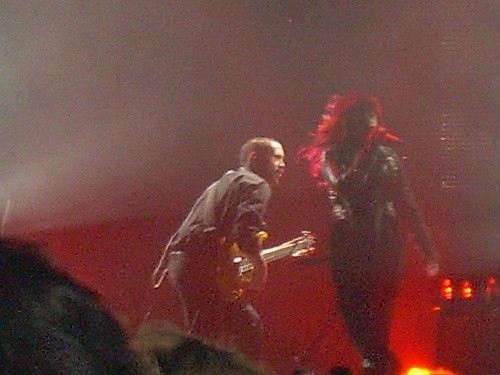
[0,241,352,374]
[302,89,443,370]
[151,137,286,359]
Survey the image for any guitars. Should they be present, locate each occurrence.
[217,227,316,293]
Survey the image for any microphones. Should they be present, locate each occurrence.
[383,131,403,145]
